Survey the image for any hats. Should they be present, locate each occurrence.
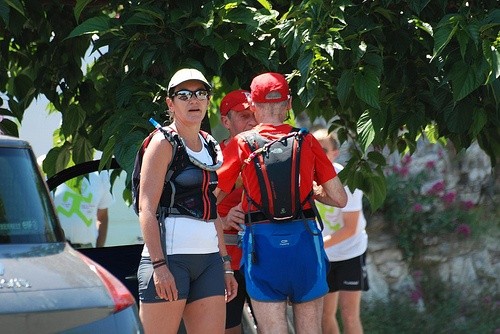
[167,68,211,97]
[220,90,256,116]
[250,72,290,103]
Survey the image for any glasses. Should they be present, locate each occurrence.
[171,89,209,101]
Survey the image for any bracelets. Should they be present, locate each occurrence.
[152,259,167,269]
[221,255,232,263]
[224,269,235,274]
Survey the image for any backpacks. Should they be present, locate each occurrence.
[132,125,218,218]
[238,127,309,221]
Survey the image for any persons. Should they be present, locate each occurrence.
[39,153,112,248]
[136,68,238,334]
[212,72,371,334]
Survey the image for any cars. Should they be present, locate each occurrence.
[0,134,159,334]
